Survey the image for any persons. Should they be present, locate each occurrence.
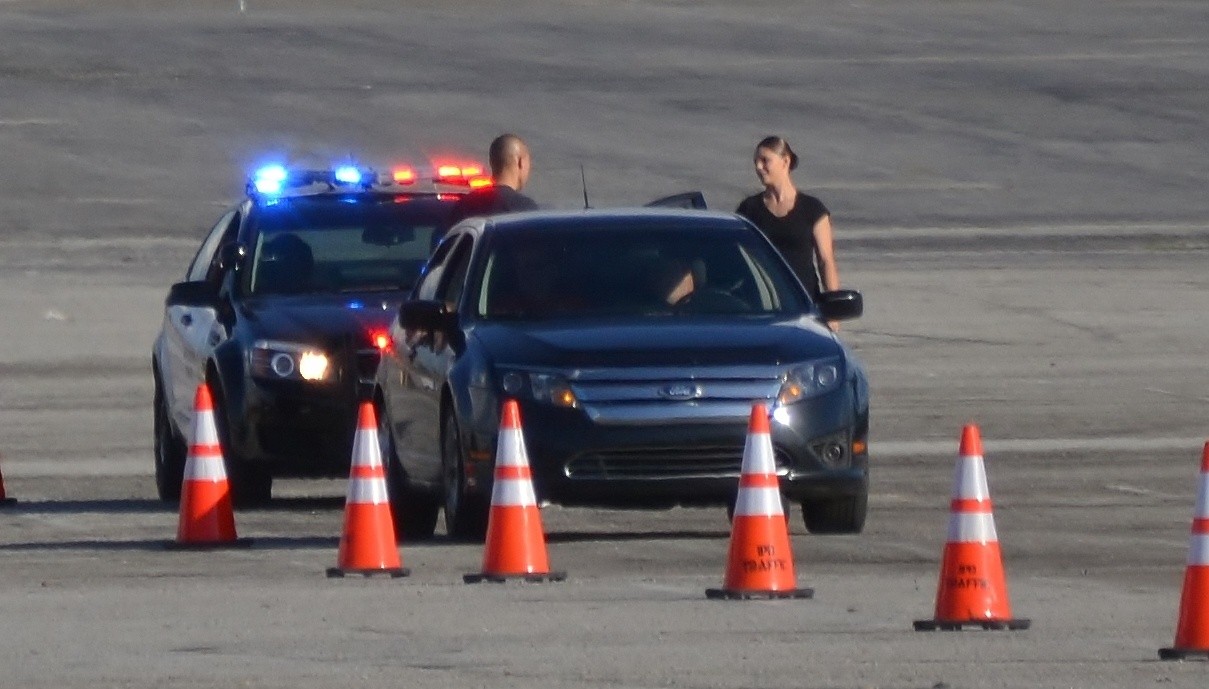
[736,135,840,334]
[453,134,538,242]
[639,248,696,321]
[267,233,327,291]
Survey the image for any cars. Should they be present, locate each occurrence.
[154,166,539,510]
[375,199,875,541]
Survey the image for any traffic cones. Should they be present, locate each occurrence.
[326,397,409,579]
[723,405,799,593]
[173,382,238,551]
[478,395,551,582]
[931,423,1017,628]
[1165,441,1208,649]
